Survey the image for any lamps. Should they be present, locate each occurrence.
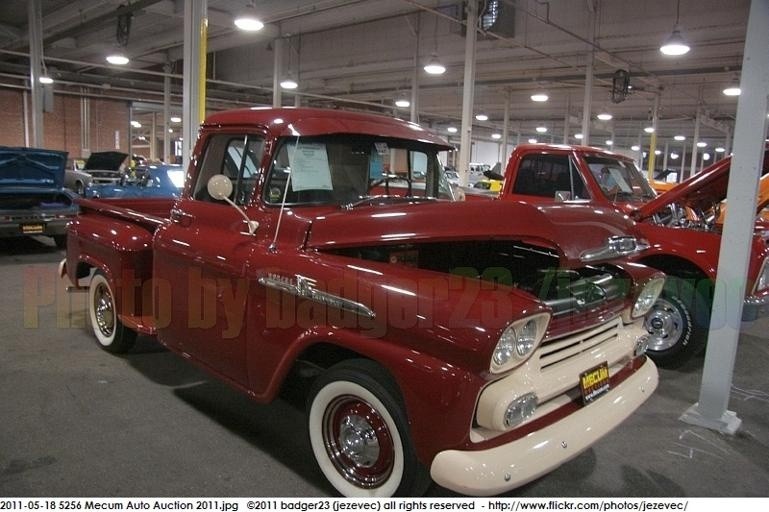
[38,0,741,160]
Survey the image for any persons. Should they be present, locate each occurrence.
[596,167,622,196]
